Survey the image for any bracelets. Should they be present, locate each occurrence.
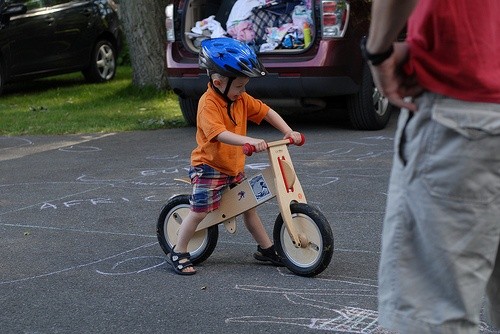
[361,34,394,66]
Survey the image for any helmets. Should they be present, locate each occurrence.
[198,38,270,80]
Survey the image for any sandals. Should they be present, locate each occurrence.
[165,244,196,275]
[253,244,287,267]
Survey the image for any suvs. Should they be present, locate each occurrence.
[162,0,409,132]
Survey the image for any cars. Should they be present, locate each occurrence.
[0,0,124,94]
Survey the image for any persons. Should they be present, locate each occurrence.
[166,38,302,275]
[361,1,500,334]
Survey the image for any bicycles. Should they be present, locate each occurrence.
[155,134,335,278]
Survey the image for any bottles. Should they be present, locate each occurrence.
[303,22,311,47]
